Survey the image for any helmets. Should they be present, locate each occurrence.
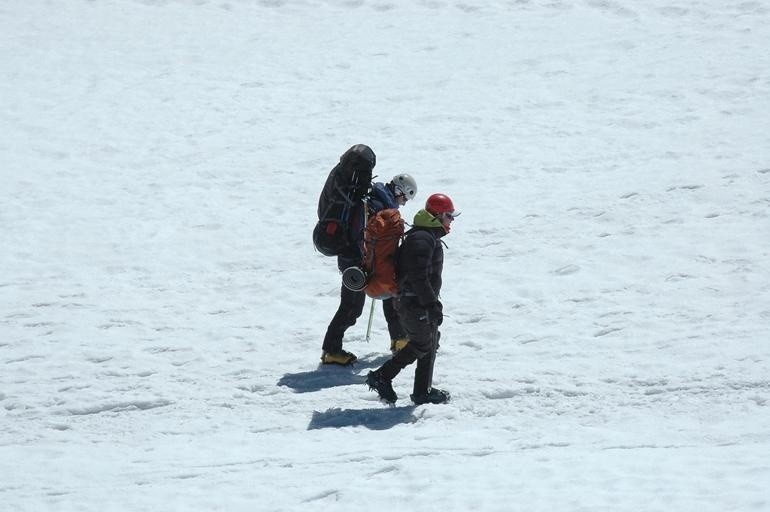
[426,194,455,220]
[393,174,417,201]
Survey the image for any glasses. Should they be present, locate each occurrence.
[445,213,452,218]
[398,188,408,202]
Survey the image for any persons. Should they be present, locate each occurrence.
[365,194,463,407]
[320,172,418,365]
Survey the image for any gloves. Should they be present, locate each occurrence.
[425,303,443,325]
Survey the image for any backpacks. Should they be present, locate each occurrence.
[313,145,396,292]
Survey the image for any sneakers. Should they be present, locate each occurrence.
[392,336,410,354]
[413,388,450,406]
[366,369,398,403]
[322,350,358,366]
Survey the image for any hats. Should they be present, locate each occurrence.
[446,209,463,218]
